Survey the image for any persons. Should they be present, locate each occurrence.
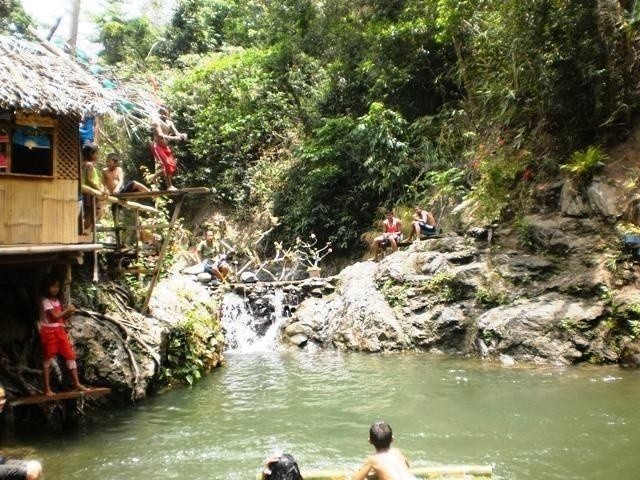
[100,151,152,214]
[196,229,229,283]
[79,141,106,234]
[35,276,92,396]
[259,453,303,480]
[350,422,416,480]
[404,203,438,246]
[366,206,404,261]
[77,111,100,144]
[151,106,189,190]
